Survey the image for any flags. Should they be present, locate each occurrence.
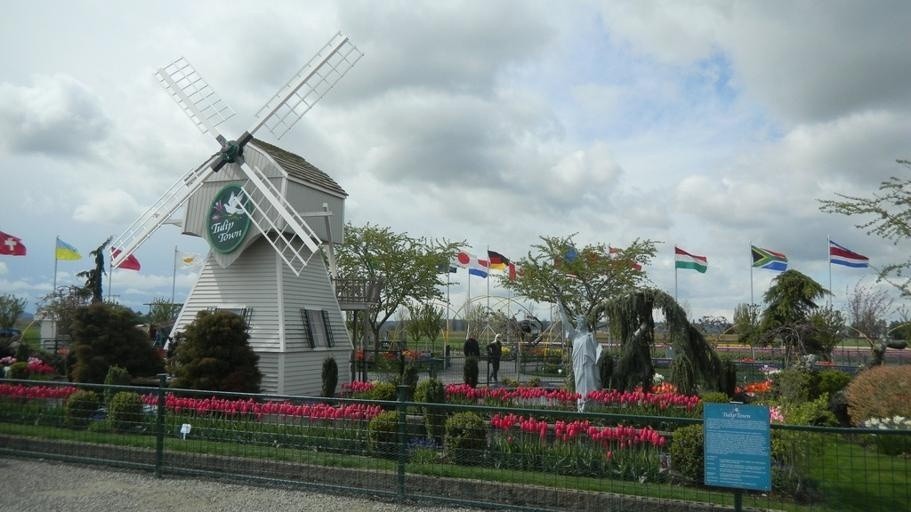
[827,238,869,269]
[174,250,202,271]
[0,231,27,256]
[111,247,140,271]
[508,262,524,281]
[469,258,489,278]
[749,243,789,272]
[55,238,80,261]
[609,247,642,271]
[488,251,509,271]
[449,249,477,270]
[674,248,710,274]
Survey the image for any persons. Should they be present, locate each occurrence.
[555,298,602,409]
[485,333,502,384]
[463,332,479,384]
